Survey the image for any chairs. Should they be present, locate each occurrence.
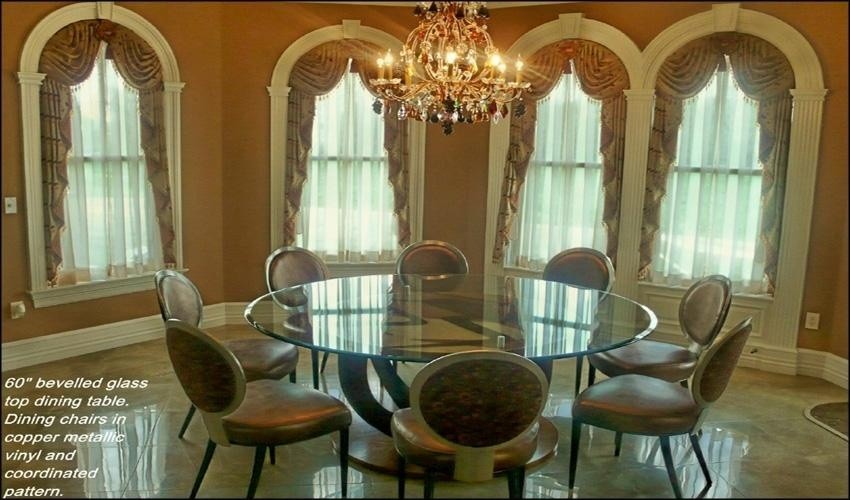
[153,239,753,498]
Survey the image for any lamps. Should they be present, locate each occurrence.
[369,2,530,136]
